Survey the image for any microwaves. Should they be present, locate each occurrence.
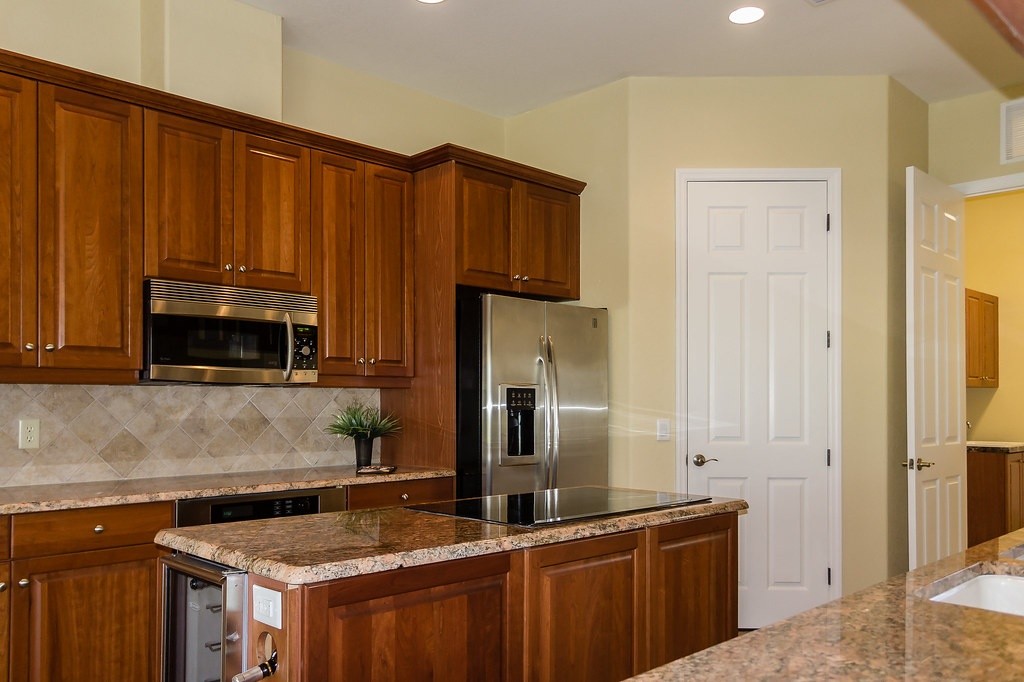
[143,278,319,384]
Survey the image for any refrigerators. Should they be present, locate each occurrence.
[460,294,608,494]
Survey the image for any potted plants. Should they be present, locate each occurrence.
[322,396,405,468]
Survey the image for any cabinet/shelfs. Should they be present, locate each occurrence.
[143,107,309,294]
[1,69,142,371]
[9,500,175,682]
[522,510,738,682]
[417,157,580,307]
[347,476,454,511]
[965,288,999,388]
[154,550,523,682]
[310,148,416,378]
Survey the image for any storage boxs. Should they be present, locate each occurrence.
[967,451,1024,548]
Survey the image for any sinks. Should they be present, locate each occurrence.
[917,559,1024,616]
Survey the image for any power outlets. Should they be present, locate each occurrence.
[19,419,41,449]
